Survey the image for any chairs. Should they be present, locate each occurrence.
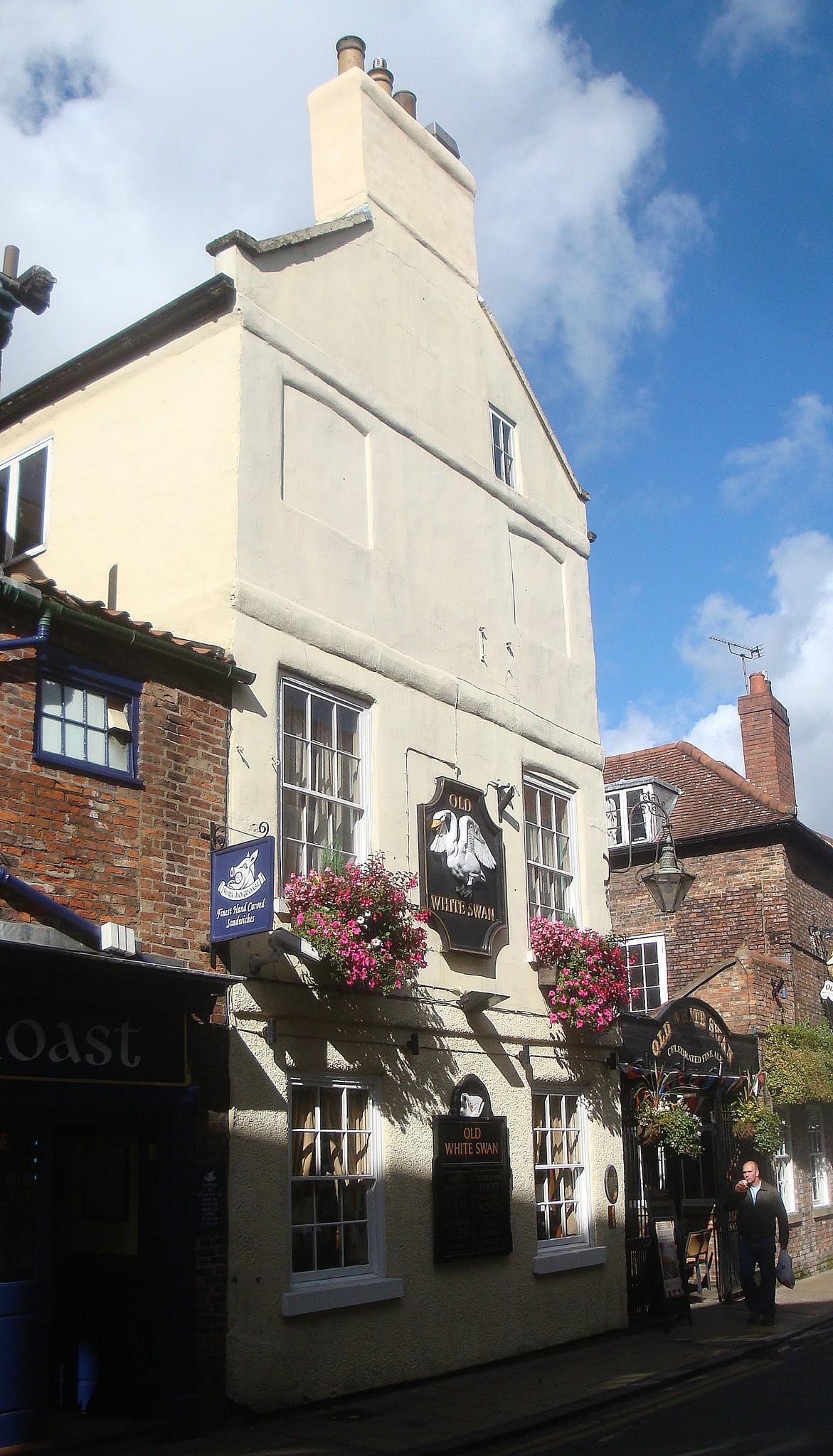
[683,1228,713,1297]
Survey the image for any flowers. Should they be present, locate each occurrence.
[283,838,432,995]
[630,1058,708,1161]
[727,1064,789,1159]
[530,909,643,1039]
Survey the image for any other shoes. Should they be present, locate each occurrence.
[747,1307,761,1324]
[761,1315,774,1327]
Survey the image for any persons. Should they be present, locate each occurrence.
[724,1160,789,1325]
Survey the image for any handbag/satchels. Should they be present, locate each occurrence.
[776,1247,795,1289]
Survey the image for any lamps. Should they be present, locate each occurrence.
[636,1052,649,1069]
[675,1057,687,1072]
[406,1033,419,1056]
[606,1052,617,1071]
[517,1045,531,1065]
[771,976,786,998]
[609,791,698,915]
[263,1018,277,1048]
[492,783,516,824]
[811,912,833,942]
[743,1066,754,1082]
[711,1062,724,1081]
[456,990,510,1013]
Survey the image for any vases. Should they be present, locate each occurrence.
[634,1123,667,1149]
[538,959,562,986]
[734,1119,758,1143]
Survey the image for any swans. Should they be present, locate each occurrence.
[429,810,497,899]
